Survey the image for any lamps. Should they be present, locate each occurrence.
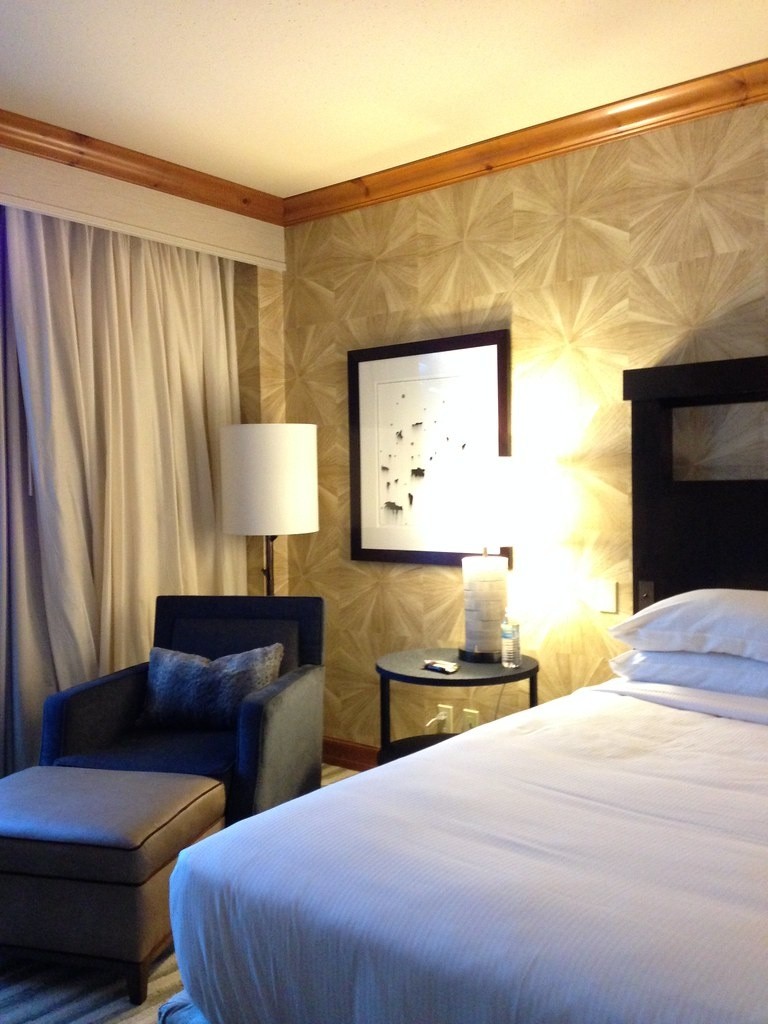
[420,451,538,663]
[220,422,320,596]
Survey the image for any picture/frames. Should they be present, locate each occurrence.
[347,328,513,569]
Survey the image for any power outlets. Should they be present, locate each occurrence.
[462,709,479,732]
[438,704,453,733]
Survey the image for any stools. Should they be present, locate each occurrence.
[0,765,225,1005]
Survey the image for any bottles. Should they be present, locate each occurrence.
[501,607,522,669]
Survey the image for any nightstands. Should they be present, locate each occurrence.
[375,646,540,765]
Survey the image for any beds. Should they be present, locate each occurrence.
[158,353,768,1024]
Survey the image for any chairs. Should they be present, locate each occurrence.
[40,596,326,827]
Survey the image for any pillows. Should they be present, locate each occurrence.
[142,642,283,745]
[607,588,768,664]
[608,649,768,699]
